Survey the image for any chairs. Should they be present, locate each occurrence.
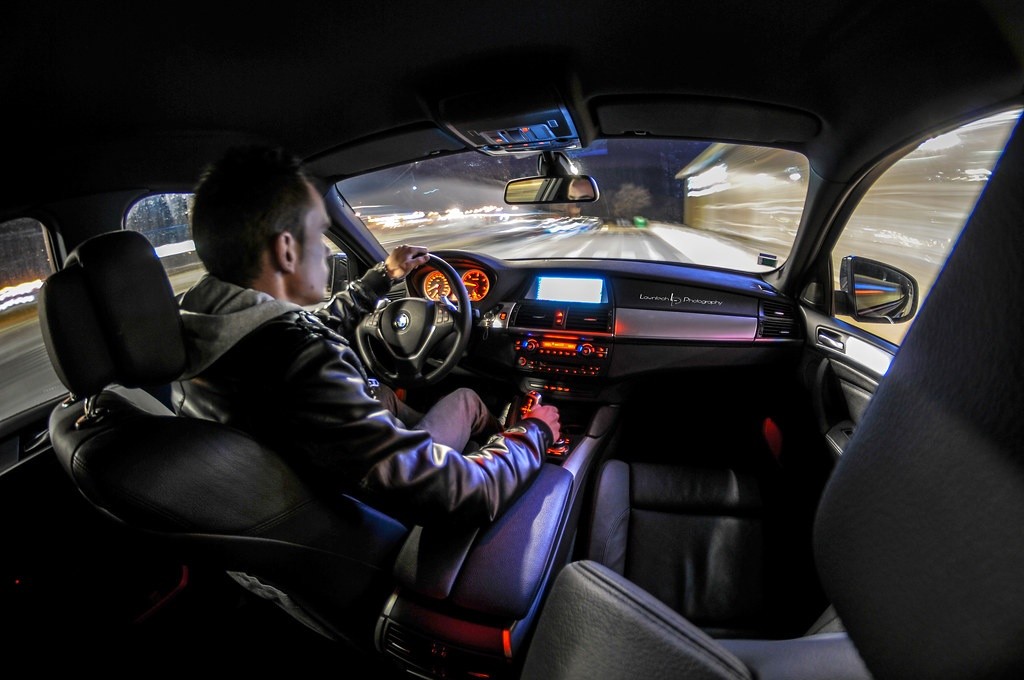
[521,108,1024,680]
[36,224,411,657]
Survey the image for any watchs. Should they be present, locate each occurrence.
[374,261,394,286]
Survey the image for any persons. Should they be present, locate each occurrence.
[170,155,560,530]
[569,178,594,200]
